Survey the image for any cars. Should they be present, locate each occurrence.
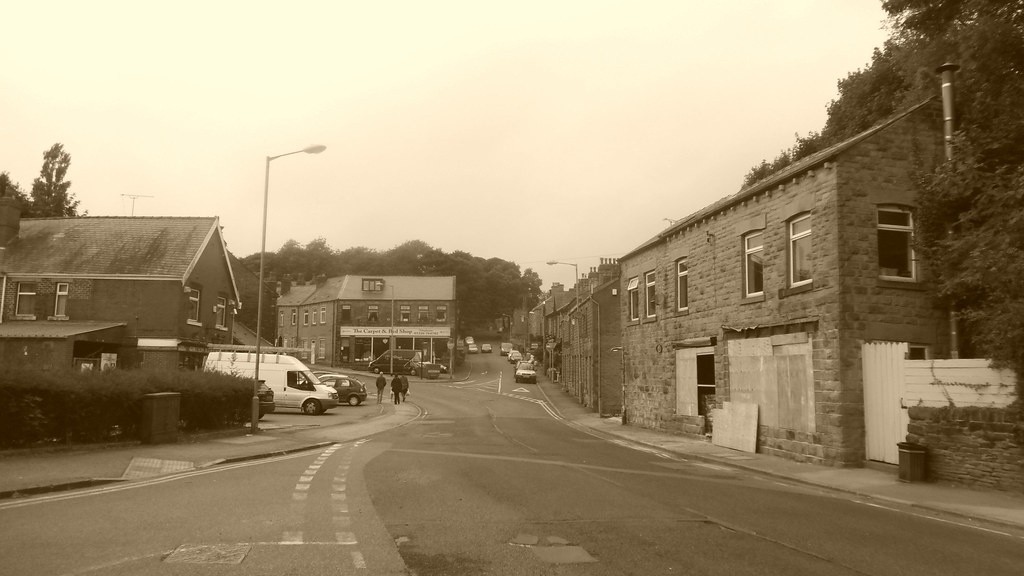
[296,372,335,387]
[507,349,523,364]
[317,375,367,407]
[468,344,479,353]
[514,361,537,383]
[465,337,475,345]
[481,344,492,352]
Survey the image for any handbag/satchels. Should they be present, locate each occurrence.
[405,389,411,397]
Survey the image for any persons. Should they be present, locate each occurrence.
[376,372,386,404]
[390,374,409,404]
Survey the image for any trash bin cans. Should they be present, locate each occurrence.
[897,442,928,483]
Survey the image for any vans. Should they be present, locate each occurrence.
[500,343,513,356]
[368,348,422,376]
[203,351,338,416]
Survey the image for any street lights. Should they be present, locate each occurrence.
[546,260,583,403]
[251,144,327,429]
[611,346,627,424]
[377,282,394,376]
